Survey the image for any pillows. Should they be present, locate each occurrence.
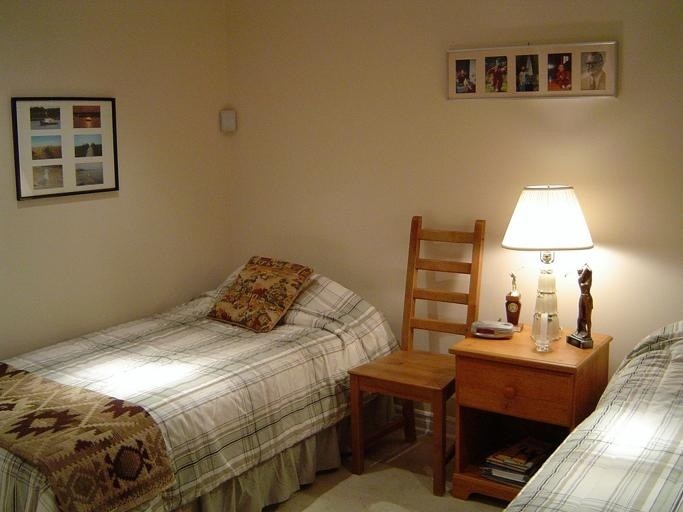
[211,254,317,333]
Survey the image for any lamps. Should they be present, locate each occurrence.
[500,183,596,342]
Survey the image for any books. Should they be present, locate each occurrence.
[477,432,553,489]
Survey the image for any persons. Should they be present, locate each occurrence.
[518,67,528,90]
[455,60,476,94]
[487,59,506,91]
[555,64,571,91]
[580,52,607,90]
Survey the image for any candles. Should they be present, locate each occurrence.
[539,312,548,341]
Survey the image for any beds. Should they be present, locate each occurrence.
[500,321,683,512]
[0,256,402,512]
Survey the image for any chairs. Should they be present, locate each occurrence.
[347,216,487,497]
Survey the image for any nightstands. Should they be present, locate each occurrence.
[448,323,614,502]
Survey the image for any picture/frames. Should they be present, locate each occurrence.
[446,41,619,101]
[10,96,120,201]
